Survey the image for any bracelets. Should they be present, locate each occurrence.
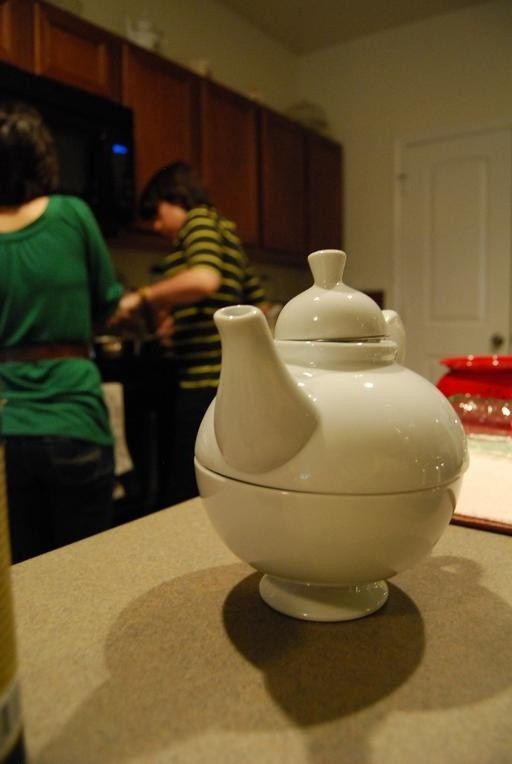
[129,285,151,307]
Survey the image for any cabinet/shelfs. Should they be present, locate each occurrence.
[1,0,120,135]
[98,40,260,251]
[258,104,344,268]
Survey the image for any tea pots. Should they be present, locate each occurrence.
[190,247,471,626]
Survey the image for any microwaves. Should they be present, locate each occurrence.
[0,60,138,235]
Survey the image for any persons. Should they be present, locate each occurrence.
[103,154,276,527]
[1,109,128,566]
